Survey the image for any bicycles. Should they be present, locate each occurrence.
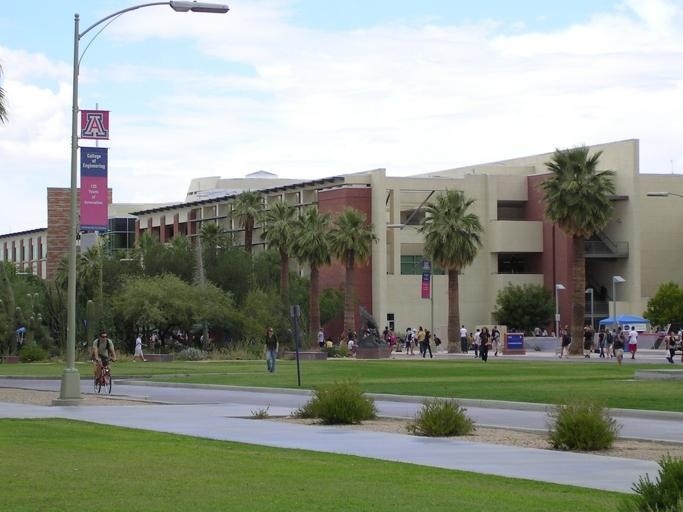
[91,357,117,394]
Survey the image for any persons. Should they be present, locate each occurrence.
[131,333,148,362]
[93,332,116,385]
[384,326,442,357]
[584,323,638,364]
[510,325,572,358]
[318,328,371,353]
[650,327,683,362]
[264,327,279,372]
[460,324,500,362]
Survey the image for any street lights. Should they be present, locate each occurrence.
[385,223,437,354]
[586,289,594,327]
[647,192,683,199]
[555,284,567,337]
[613,276,626,324]
[50,0,229,406]
